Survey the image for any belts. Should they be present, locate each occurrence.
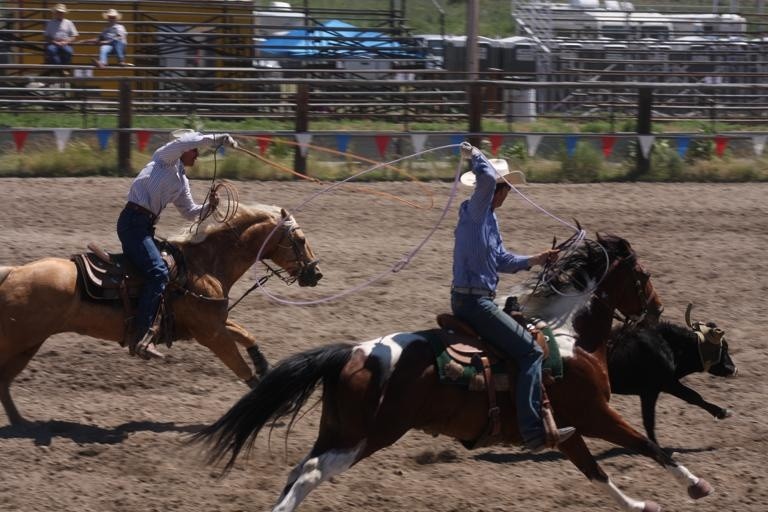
[125,201,160,225]
[451,286,496,297]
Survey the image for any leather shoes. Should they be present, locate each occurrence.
[134,340,165,361]
[521,427,576,453]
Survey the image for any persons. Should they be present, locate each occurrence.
[91,7,135,70]
[427,142,579,457]
[43,3,80,77]
[113,127,239,363]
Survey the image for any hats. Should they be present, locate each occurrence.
[102,8,121,21]
[168,127,194,139]
[50,3,68,13]
[460,158,528,186]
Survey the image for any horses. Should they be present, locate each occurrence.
[0,201,324,428]
[175,216,716,512]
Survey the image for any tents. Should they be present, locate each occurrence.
[252,17,430,63]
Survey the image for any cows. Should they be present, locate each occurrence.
[606,300,738,445]
